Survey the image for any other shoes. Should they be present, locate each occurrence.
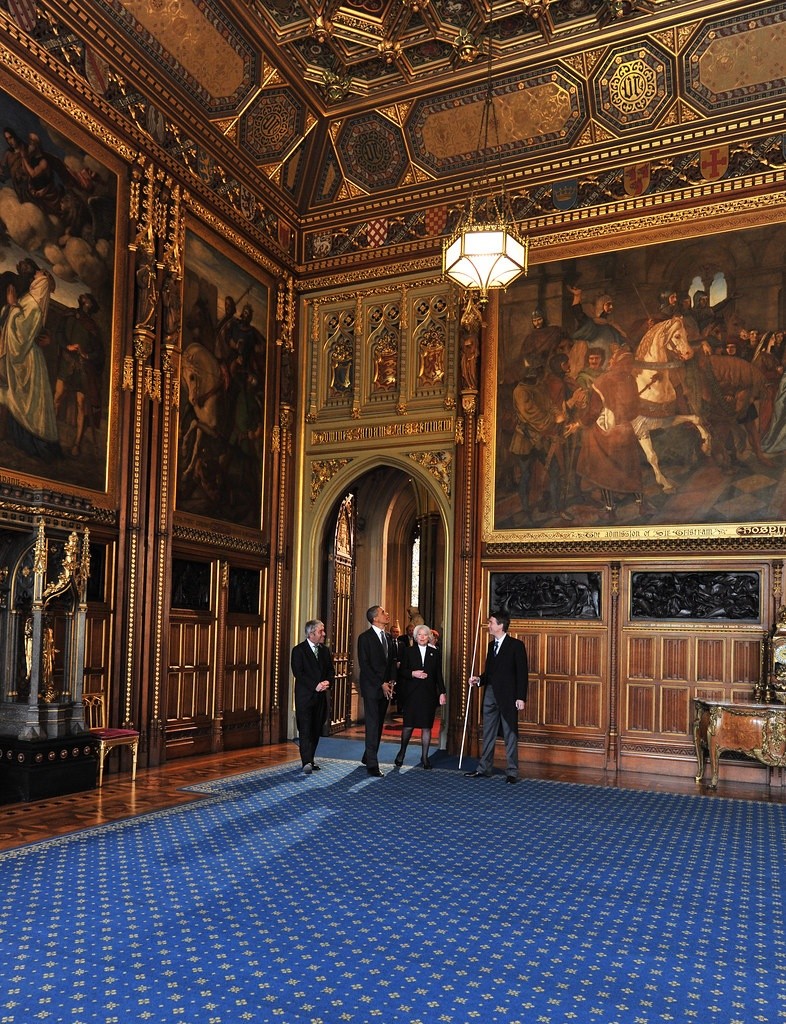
[303,763,313,774]
[313,764,321,770]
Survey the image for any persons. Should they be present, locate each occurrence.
[394,625,447,769]
[464,611,528,785]
[390,625,415,714]
[428,630,440,648]
[291,620,335,774]
[357,605,398,777]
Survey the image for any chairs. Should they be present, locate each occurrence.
[83,696,140,787]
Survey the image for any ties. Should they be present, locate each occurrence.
[494,641,499,657]
[380,631,388,661]
[393,639,398,659]
[314,646,319,663]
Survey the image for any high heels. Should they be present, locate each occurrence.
[395,751,405,766]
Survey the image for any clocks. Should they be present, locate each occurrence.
[753,605,786,704]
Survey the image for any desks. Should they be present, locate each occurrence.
[692,698,786,788]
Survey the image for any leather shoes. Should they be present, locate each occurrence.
[420,758,433,771]
[367,769,384,777]
[464,770,492,779]
[361,759,367,764]
[505,776,517,785]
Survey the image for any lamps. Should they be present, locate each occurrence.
[440,1,530,313]
